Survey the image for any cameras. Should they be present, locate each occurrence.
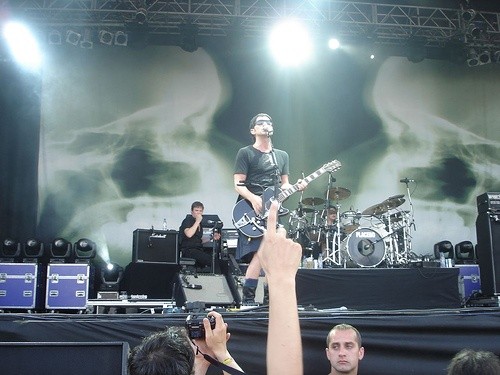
[186,313,216,339]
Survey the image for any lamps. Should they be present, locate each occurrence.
[466,23,481,39]
[75,238,97,259]
[1,238,22,258]
[461,8,477,23]
[454,241,473,260]
[466,45,500,67]
[48,238,72,257]
[135,9,148,26]
[433,242,455,260]
[50,30,130,49]
[23,240,45,258]
[102,261,123,284]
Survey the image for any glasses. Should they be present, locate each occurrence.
[253,120,271,128]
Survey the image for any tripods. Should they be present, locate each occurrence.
[293,184,420,268]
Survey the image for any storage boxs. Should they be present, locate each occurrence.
[98,292,120,300]
[45,263,90,309]
[1,262,38,309]
[454,265,481,306]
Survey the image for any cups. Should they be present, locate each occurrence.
[306,258,314,269]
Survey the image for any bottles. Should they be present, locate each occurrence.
[318,254,323,269]
[440,252,445,268]
[163,219,167,231]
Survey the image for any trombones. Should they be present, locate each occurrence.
[231,159,342,239]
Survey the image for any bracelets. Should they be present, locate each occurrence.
[222,358,234,364]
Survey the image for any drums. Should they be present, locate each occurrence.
[304,225,322,241]
[288,227,322,260]
[339,210,361,233]
[340,226,387,268]
[320,224,339,235]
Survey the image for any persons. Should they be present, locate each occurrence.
[256,200,306,375]
[325,324,364,375]
[179,202,222,268]
[126,311,246,375]
[232,113,309,307]
[448,349,500,375]
[320,205,340,232]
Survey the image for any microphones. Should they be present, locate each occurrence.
[330,175,336,182]
[207,219,223,224]
[184,284,202,290]
[400,179,415,183]
[263,126,273,132]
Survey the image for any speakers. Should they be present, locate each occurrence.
[132,228,180,264]
[171,271,236,310]
[475,212,500,295]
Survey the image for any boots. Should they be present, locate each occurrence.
[242,286,257,306]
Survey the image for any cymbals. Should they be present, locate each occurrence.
[324,186,351,200]
[294,207,315,213]
[375,210,411,225]
[302,197,325,206]
[316,210,337,216]
[362,194,406,218]
